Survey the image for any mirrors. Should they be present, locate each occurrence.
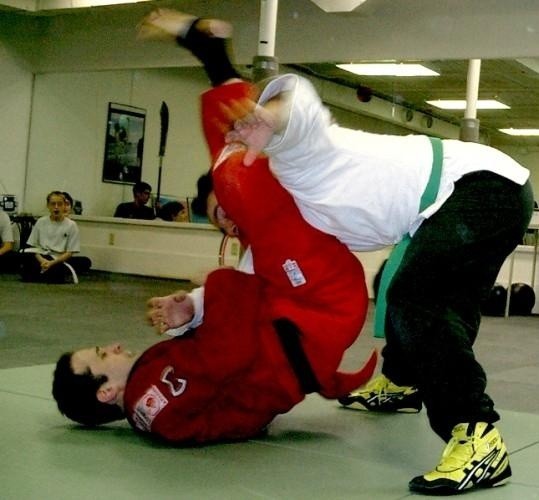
[22,53,539,253]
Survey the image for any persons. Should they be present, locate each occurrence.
[17,189,90,283]
[158,202,188,224]
[190,71,537,494]
[61,193,74,217]
[112,181,156,220]
[50,8,378,447]
[1,208,22,276]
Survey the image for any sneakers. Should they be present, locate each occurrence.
[409,421,511,495]
[338,374,423,413]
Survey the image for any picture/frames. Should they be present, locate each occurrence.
[102,102,148,186]
